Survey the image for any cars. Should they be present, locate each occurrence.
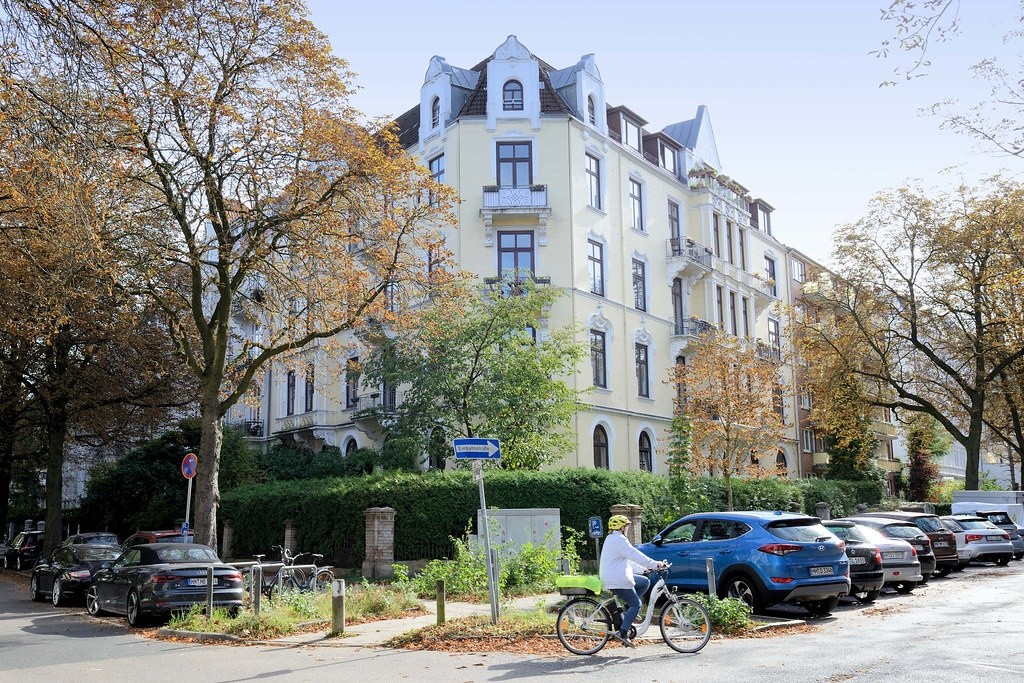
[855,524,923,595]
[952,511,1024,560]
[0,540,10,563]
[87,543,243,627]
[820,519,884,601]
[30,544,124,608]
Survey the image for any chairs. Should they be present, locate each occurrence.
[709,524,726,540]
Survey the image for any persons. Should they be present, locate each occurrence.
[598,515,664,650]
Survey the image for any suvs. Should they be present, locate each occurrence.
[635,509,851,617]
[848,512,960,577]
[119,527,194,551]
[939,515,1014,567]
[61,531,123,549]
[4,530,45,571]
[831,516,936,586]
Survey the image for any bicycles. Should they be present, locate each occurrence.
[269,548,335,601]
[555,558,712,656]
[239,544,306,596]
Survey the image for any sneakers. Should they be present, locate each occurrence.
[615,629,635,649]
[622,603,642,620]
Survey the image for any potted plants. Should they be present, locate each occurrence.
[688,167,752,203]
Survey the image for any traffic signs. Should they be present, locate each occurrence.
[454,438,501,460]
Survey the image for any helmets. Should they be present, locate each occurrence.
[608,515,632,529]
